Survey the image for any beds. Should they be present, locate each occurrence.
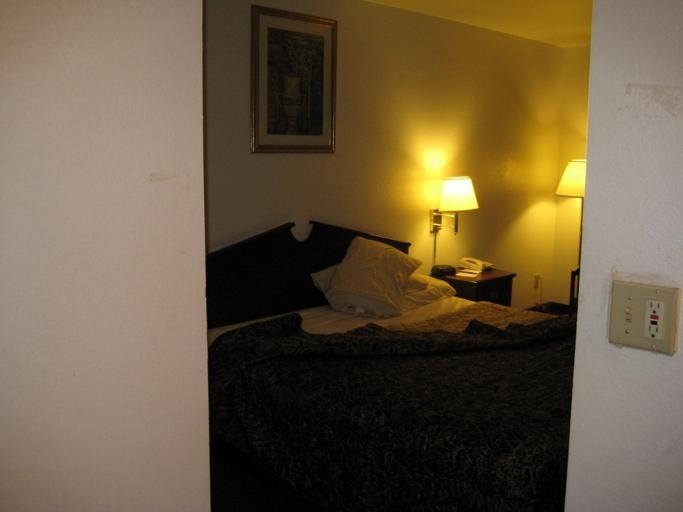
[205,218,570,512]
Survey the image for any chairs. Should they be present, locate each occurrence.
[525,268,580,314]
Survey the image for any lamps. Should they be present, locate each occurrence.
[432,176,479,234]
[555,159,587,266]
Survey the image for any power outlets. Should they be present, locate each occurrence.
[608,280,680,356]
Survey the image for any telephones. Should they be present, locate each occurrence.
[461,257,495,272]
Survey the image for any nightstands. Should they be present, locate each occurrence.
[430,264,517,307]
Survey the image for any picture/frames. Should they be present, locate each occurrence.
[250,4,336,154]
